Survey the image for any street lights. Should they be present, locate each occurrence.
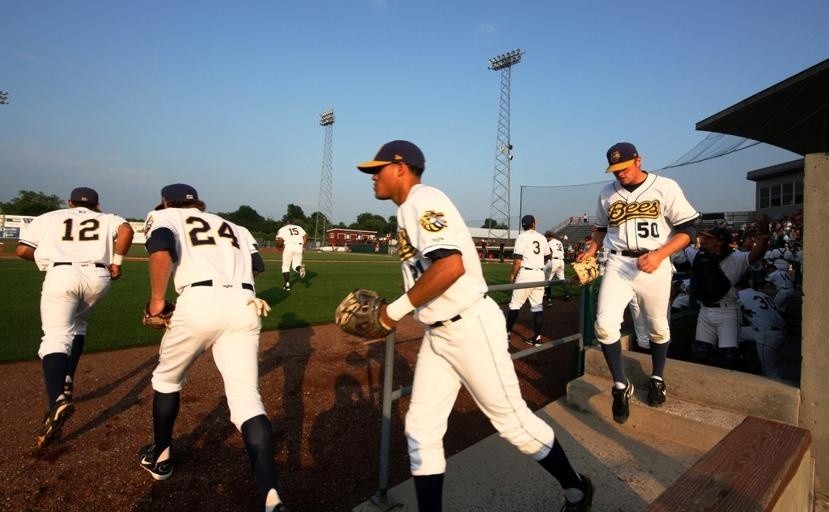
[313,108,336,248]
[0,89,11,106]
[484,47,528,253]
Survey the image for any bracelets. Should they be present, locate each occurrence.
[383,291,417,323]
[111,252,125,267]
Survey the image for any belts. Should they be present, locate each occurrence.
[53,261,107,268]
[430,314,462,327]
[192,280,254,291]
[554,257,558,259]
[525,266,544,271]
[610,249,648,258]
[703,302,720,307]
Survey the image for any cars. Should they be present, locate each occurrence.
[0,226,20,237]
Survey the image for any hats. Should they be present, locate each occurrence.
[605,141,638,173]
[70,187,100,204]
[699,227,730,239]
[155,183,206,210]
[357,140,425,176]
[522,215,534,228]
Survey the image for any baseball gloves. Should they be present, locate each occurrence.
[571,257,600,285]
[143,302,175,327]
[334,288,394,338]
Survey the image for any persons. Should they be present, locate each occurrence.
[333,139,596,510]
[603,234,679,355]
[136,183,290,512]
[575,142,700,426]
[668,213,801,381]
[16,186,135,448]
[541,212,607,306]
[274,216,310,292]
[504,213,551,348]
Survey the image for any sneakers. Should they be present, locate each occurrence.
[299,264,306,278]
[64,374,74,398]
[561,471,594,512]
[283,282,290,291]
[508,332,511,342]
[138,443,174,482]
[523,338,542,347]
[34,392,75,450]
[612,377,635,424]
[648,377,667,406]
[263,488,284,511]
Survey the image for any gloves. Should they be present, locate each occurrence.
[246,296,271,318]
